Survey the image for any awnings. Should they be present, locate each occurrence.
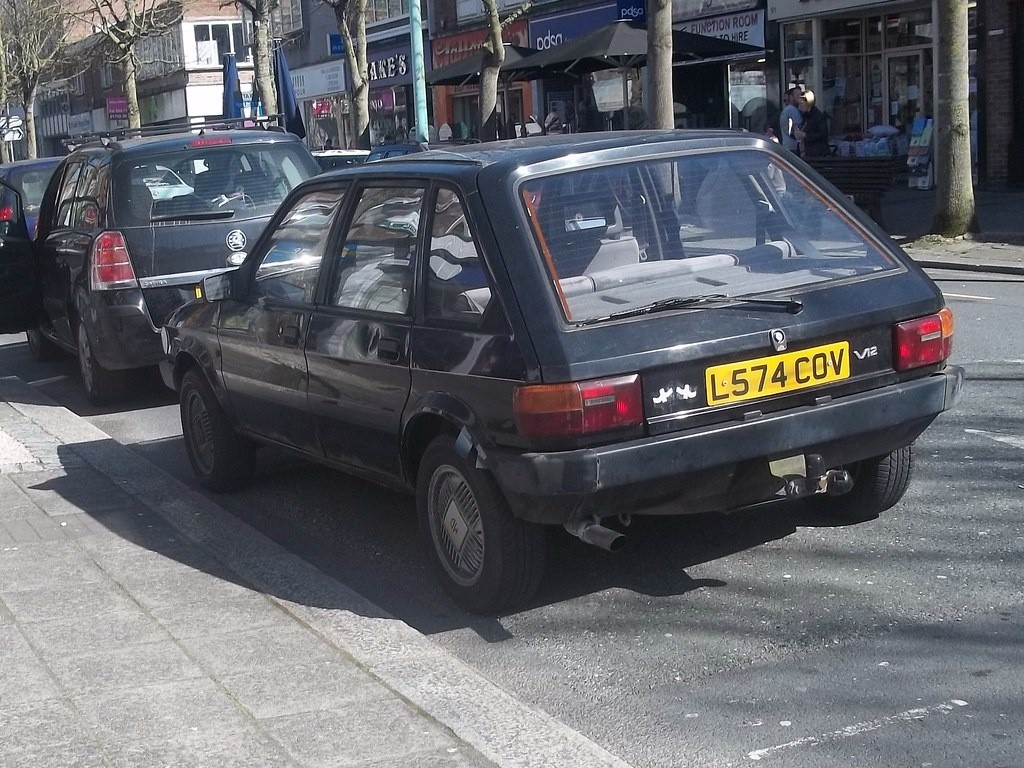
[590,77,643,113]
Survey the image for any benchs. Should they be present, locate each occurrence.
[795,155,894,231]
[453,238,798,311]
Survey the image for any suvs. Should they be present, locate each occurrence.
[363,138,481,162]
[26,114,338,405]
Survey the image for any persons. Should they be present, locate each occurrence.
[396,116,409,140]
[780,86,804,157]
[324,139,333,149]
[577,101,594,133]
[696,123,786,238]
[787,90,831,157]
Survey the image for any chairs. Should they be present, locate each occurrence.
[547,196,639,280]
[403,201,490,313]
[131,185,153,220]
[195,152,243,193]
[234,168,268,207]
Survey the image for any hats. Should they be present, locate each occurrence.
[798,89,815,106]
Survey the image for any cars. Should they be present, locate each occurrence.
[135,165,195,201]
[0,156,66,271]
[310,150,372,173]
[161,129,967,614]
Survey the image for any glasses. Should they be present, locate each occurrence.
[800,90,814,96]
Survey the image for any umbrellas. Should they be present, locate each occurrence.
[425,46,543,143]
[223,55,244,119]
[499,17,769,130]
[274,40,305,140]
